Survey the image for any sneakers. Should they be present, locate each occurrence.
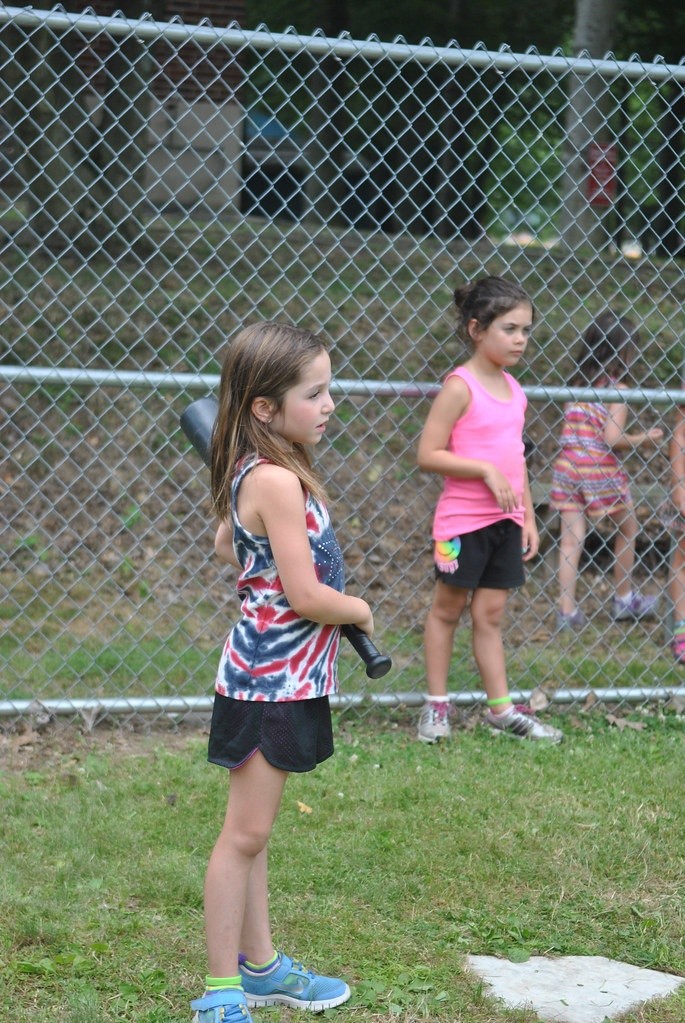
[415,700,452,745]
[671,630,685,663]
[555,603,585,633]
[611,589,661,622]
[238,951,351,1012]
[189,985,253,1023]
[481,703,564,749]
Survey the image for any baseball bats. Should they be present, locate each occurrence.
[180,396,393,679]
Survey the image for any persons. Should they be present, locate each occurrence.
[416,275,565,745]
[190,320,373,1022]
[549,313,663,632]
[660,379,685,665]
